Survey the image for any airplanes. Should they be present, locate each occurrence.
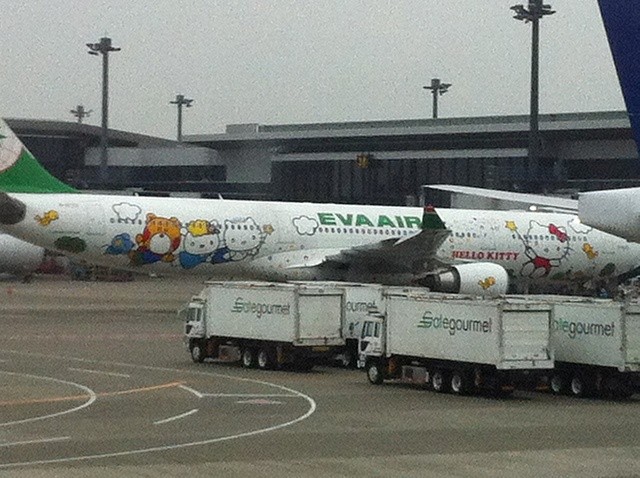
[0,119,640,295]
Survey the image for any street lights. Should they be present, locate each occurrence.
[423,78,451,118]
[511,0,555,183]
[88,36,121,187]
[72,105,93,121]
[170,94,193,142]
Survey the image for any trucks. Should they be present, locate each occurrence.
[551,301,638,397]
[178,281,345,369]
[357,296,554,397]
[335,282,430,369]
